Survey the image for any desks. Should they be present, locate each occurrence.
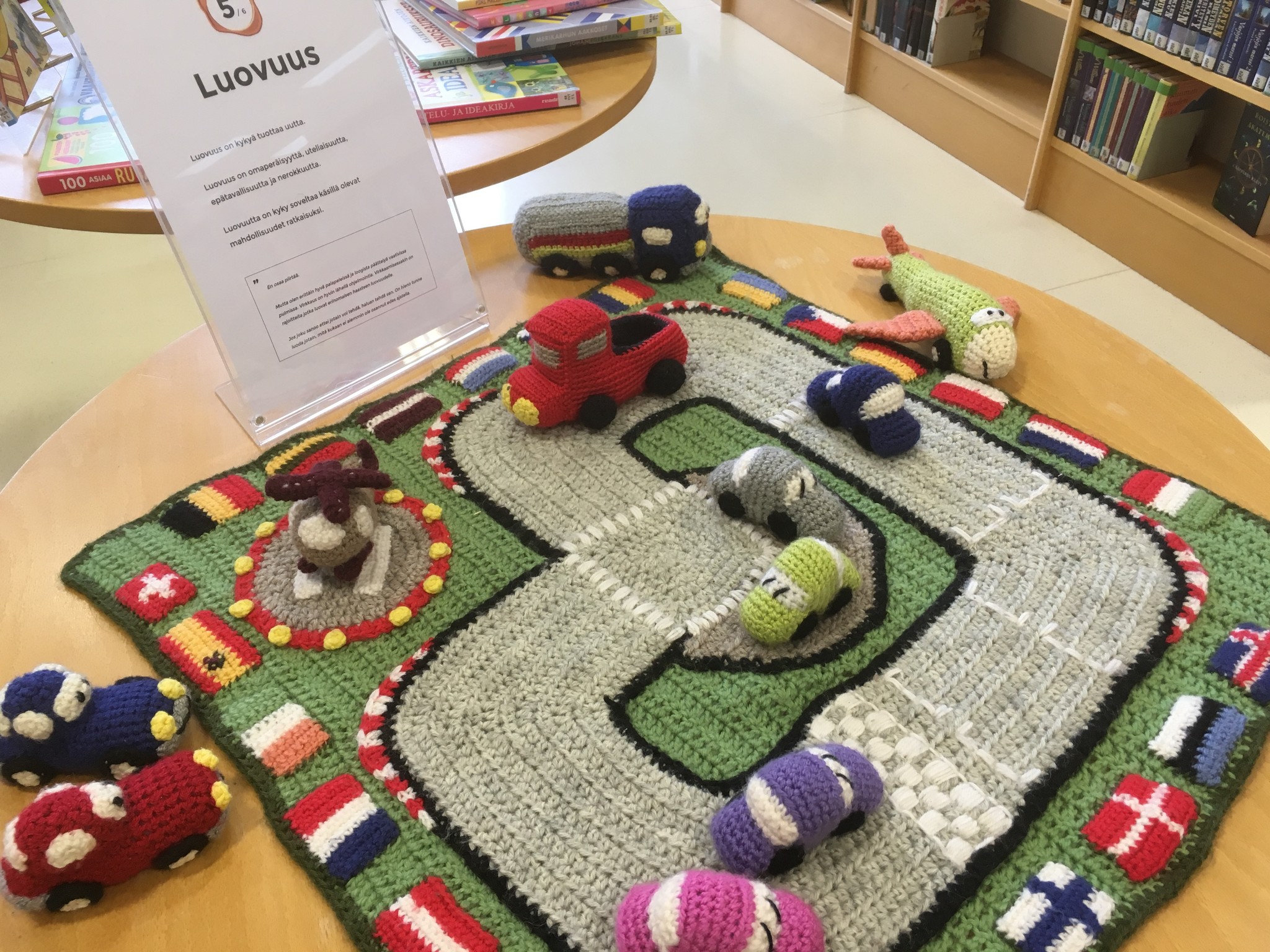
[1,216,1270,952]
[0,37,660,234]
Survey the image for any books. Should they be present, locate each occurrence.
[376,0,682,125]
[38,57,150,195]
[861,0,1270,236]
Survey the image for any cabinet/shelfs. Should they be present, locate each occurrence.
[708,0,1270,355]
